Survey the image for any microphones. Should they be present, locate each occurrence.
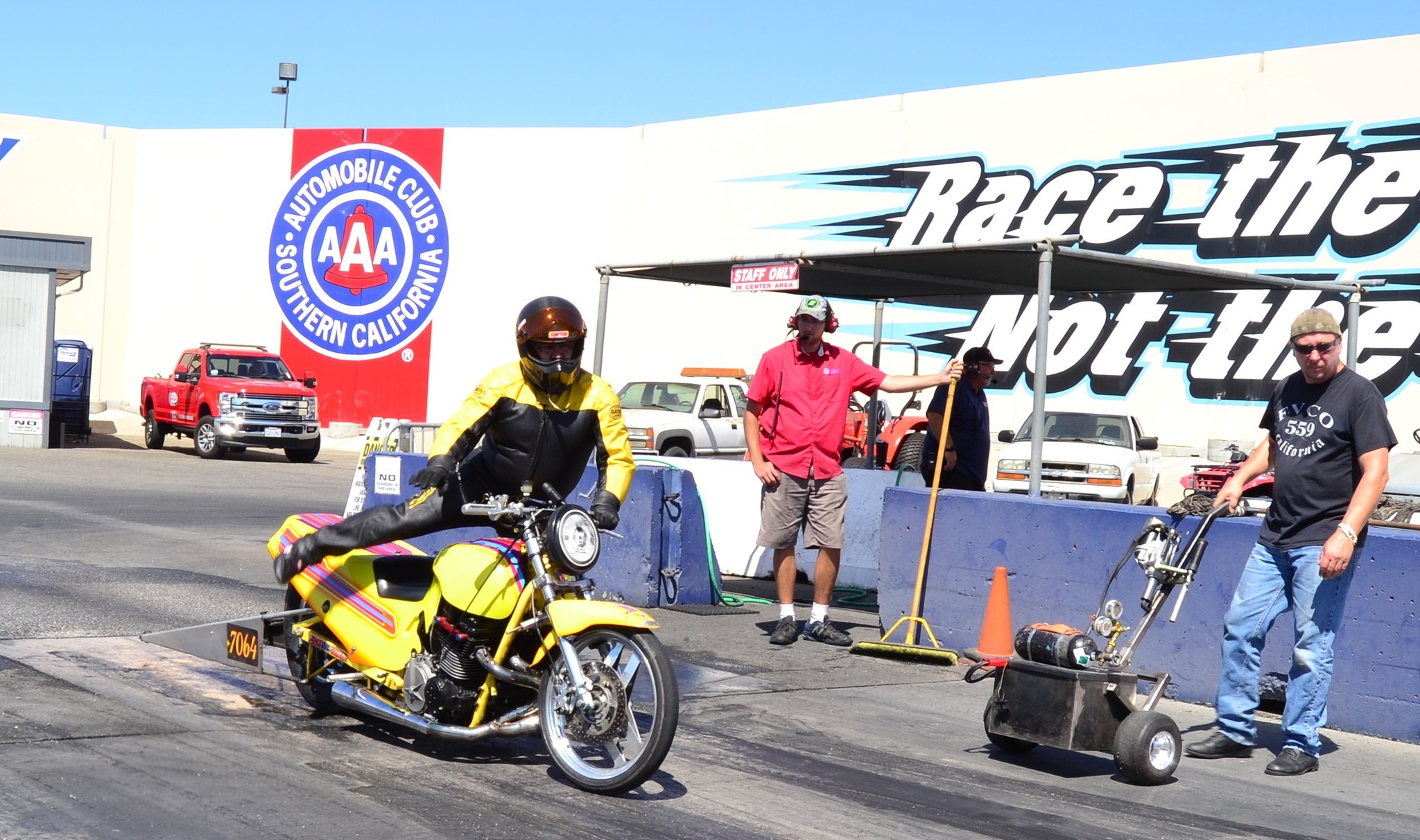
[969,367,998,384]
[791,331,809,341]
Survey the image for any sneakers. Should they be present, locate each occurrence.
[802,614,853,646]
[769,615,798,644]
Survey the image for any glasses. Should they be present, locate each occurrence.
[1291,334,1340,354]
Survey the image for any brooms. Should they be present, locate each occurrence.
[849,360,965,666]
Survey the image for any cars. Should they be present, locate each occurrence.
[992,409,1164,504]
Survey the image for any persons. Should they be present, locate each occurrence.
[1183,307,1398,777]
[744,294,965,644]
[274,294,634,585]
[922,348,1005,491]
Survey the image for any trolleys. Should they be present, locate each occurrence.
[980,489,1250,789]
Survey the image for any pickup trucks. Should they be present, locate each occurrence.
[139,341,322,465]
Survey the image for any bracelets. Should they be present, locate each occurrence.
[1336,522,1359,547]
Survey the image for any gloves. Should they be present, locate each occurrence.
[408,454,454,497]
[590,490,621,531]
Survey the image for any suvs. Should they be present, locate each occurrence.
[614,367,753,461]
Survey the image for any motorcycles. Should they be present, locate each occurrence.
[134,479,680,794]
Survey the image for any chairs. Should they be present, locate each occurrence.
[1047,425,1068,437]
[1101,425,1125,441]
[702,399,723,413]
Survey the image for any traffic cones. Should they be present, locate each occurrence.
[961,568,1024,666]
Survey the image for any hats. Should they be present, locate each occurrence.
[963,347,1005,365]
[1290,306,1342,340]
[795,294,827,321]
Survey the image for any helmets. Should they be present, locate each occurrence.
[516,295,587,393]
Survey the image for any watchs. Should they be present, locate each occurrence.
[945,443,957,454]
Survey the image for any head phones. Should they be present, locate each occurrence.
[965,347,981,378]
[788,295,838,333]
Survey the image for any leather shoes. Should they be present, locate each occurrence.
[1265,748,1319,776]
[1185,732,1258,759]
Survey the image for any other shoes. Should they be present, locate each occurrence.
[273,542,310,584]
